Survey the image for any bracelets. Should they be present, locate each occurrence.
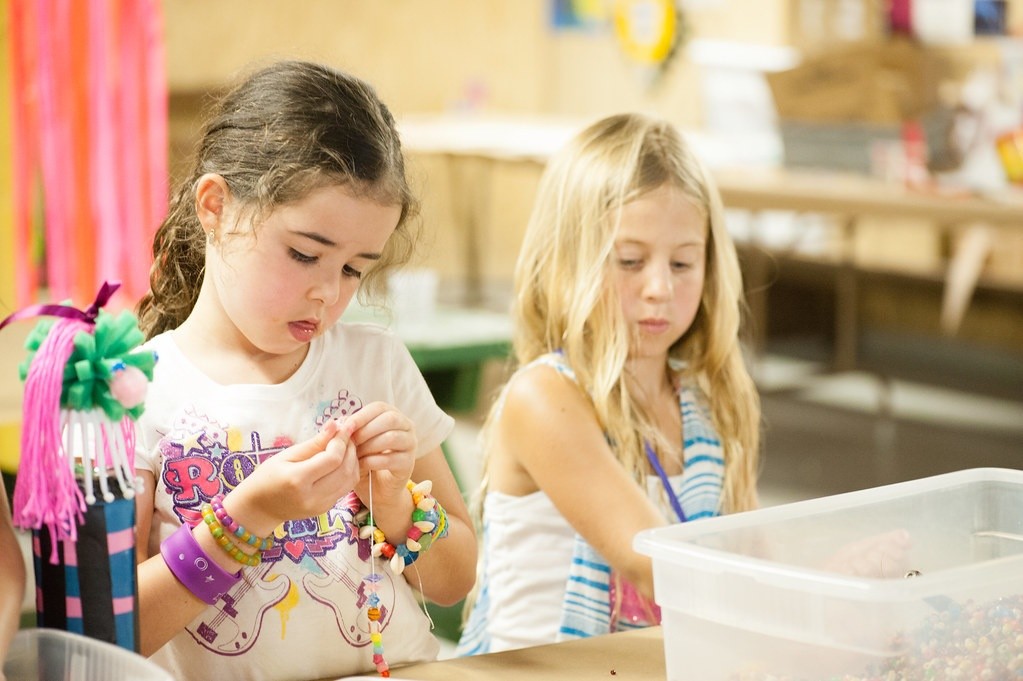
[203,493,275,566]
[349,477,448,575]
[159,522,244,605]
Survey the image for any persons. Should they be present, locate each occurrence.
[129,61,478,681]
[456,113,761,658]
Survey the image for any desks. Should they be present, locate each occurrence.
[385,112,1023,375]
[335,624,662,681]
[339,291,516,419]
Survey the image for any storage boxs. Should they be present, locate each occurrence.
[631,465,1023,681]
